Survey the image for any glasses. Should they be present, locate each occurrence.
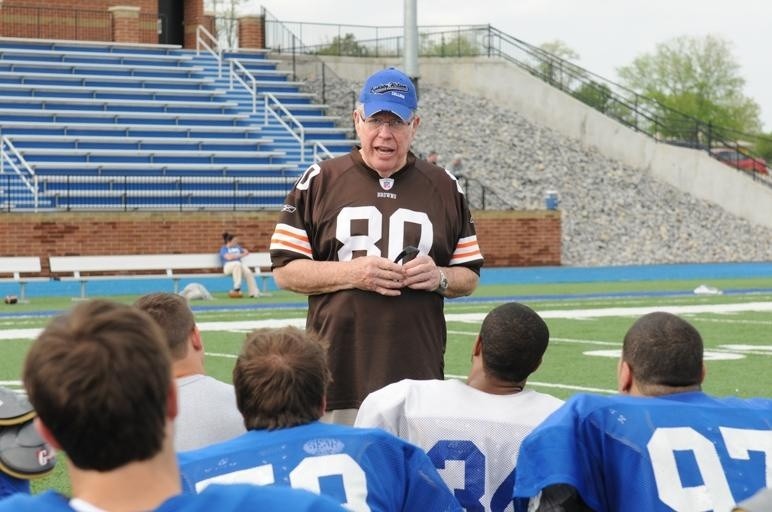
[394,246,420,264]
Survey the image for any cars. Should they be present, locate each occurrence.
[704,142,768,176]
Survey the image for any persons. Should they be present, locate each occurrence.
[129,291,249,452]
[0,299,354,511]
[268,66,485,428]
[422,150,439,165]
[353,301,568,512]
[218,232,262,298]
[445,154,470,189]
[514,310,771,511]
[175,324,469,511]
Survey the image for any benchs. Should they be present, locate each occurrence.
[0,35,364,210]
[0,252,272,304]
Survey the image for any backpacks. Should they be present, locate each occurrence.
[5,295,18,304]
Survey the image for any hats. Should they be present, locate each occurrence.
[358,67,417,123]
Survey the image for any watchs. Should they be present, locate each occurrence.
[436,267,448,296]
